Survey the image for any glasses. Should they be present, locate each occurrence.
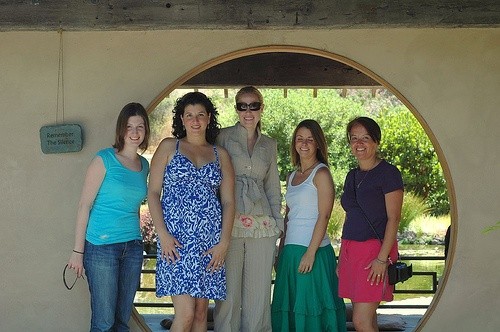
[237,102,262,111]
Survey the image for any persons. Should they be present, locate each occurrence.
[147,92,237,332]
[213,86,284,332]
[68,102,150,332]
[336,117,404,332]
[271,120,347,332]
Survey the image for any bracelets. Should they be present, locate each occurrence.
[73,250,84,254]
[377,258,387,264]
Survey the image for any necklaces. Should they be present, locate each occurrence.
[301,160,319,175]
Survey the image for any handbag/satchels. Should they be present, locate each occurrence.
[388,261,413,285]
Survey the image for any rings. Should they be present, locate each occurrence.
[378,275,380,276]
